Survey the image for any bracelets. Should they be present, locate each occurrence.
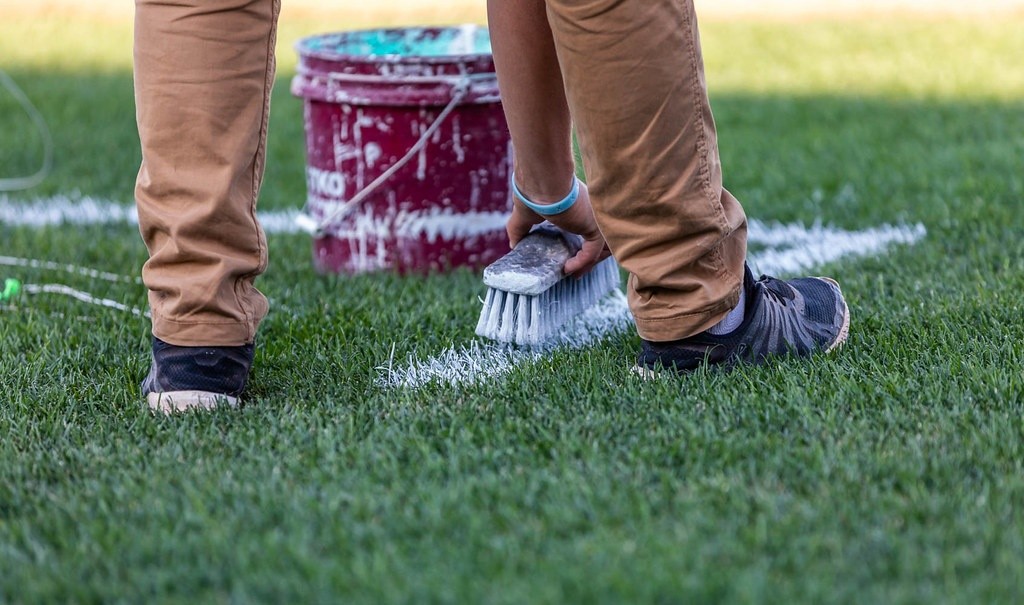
[511,174,580,215]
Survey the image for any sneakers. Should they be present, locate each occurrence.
[630,261,850,381]
[140,337,255,414]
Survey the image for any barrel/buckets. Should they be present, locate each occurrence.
[291,23,533,276]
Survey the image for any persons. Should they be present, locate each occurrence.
[132,0,851,418]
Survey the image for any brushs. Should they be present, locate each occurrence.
[474,224,621,341]
